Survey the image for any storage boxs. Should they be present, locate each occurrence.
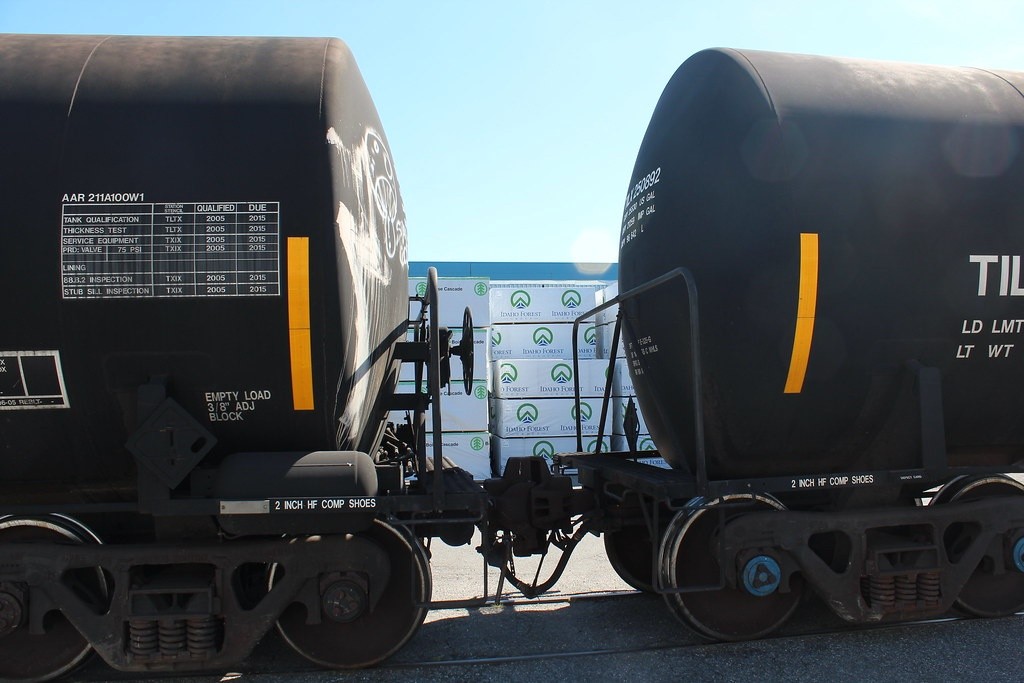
[407,277,674,481]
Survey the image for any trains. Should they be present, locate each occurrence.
[0,32,1024,683]
